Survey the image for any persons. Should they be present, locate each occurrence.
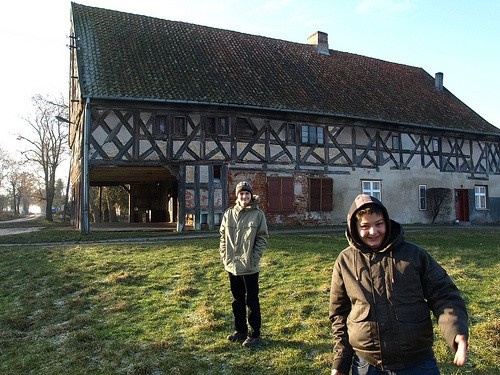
[219,180,270,346]
[329,193,470,375]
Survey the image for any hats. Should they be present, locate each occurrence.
[235,181,254,197]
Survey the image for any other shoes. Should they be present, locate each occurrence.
[242,333,262,348]
[226,329,248,341]
